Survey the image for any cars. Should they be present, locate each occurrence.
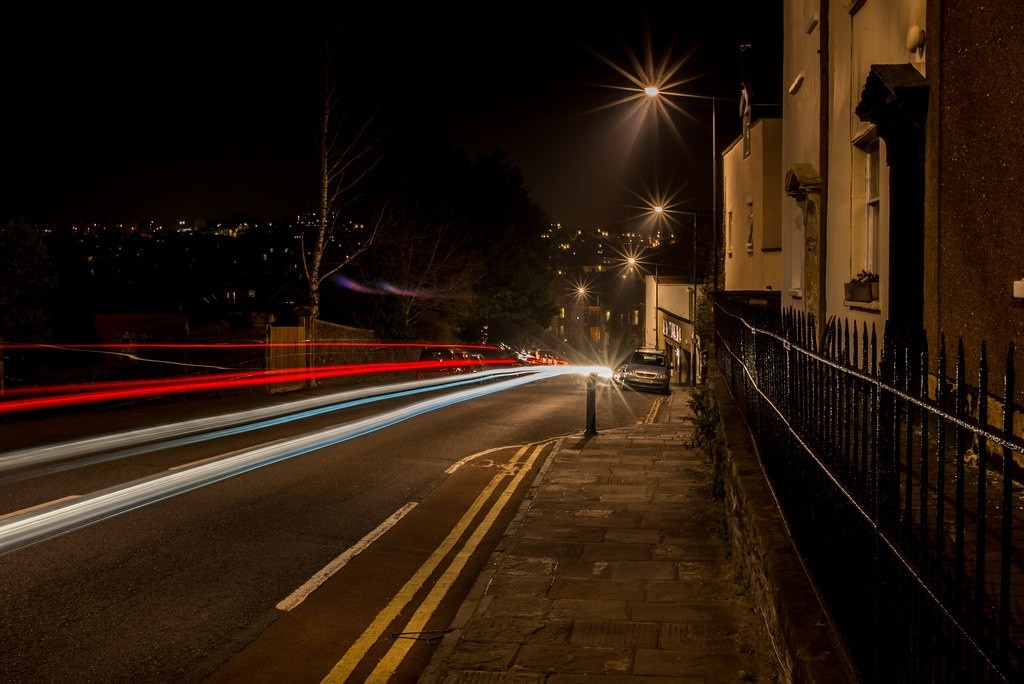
[416,345,559,379]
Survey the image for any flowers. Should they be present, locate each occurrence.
[849,266,880,283]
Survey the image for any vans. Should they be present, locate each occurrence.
[622,350,668,393]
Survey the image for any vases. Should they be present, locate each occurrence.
[842,283,881,302]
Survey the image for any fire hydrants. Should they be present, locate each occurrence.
[583,375,599,436]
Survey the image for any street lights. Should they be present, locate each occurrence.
[645,86,718,290]
[655,206,696,387]
